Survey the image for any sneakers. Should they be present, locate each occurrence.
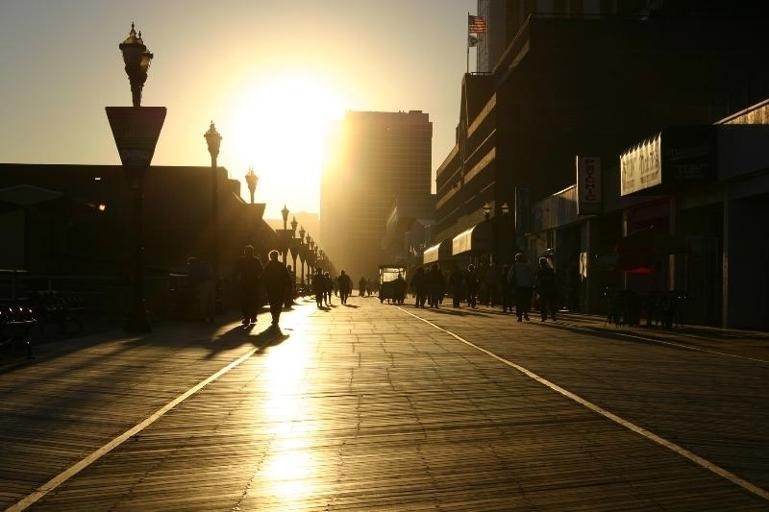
[517,314,557,322]
[242,318,257,324]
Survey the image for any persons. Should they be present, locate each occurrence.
[177,243,296,325]
[507,253,532,321]
[411,263,512,313]
[312,269,352,307]
[358,277,373,297]
[393,274,404,304]
[535,257,557,321]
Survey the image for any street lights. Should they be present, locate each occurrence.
[239,166,265,244]
[204,120,222,323]
[106,24,166,333]
[276,204,336,298]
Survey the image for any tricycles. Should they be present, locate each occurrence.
[378,265,408,304]
